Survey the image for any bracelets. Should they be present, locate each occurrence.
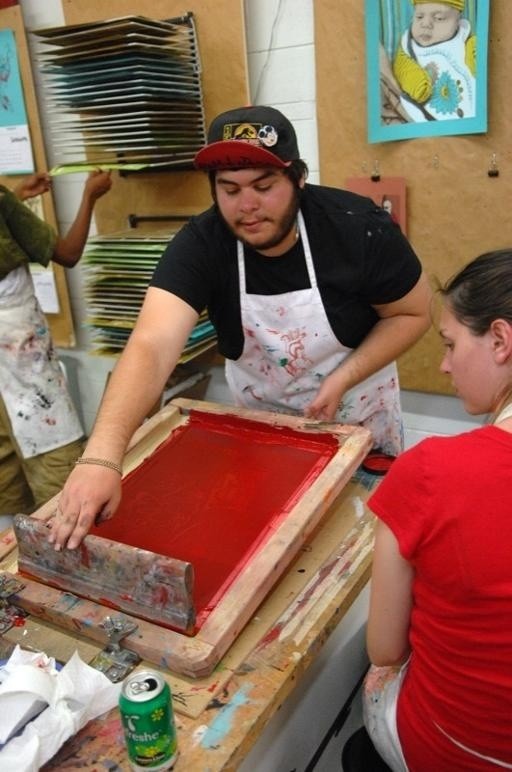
[69,454,126,477]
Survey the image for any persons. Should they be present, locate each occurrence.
[44,103,440,556]
[388,1,482,126]
[360,245,512,770]
[0,164,116,521]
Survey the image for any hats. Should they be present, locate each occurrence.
[190,103,302,175]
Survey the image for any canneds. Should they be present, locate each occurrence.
[119,672,177,772]
